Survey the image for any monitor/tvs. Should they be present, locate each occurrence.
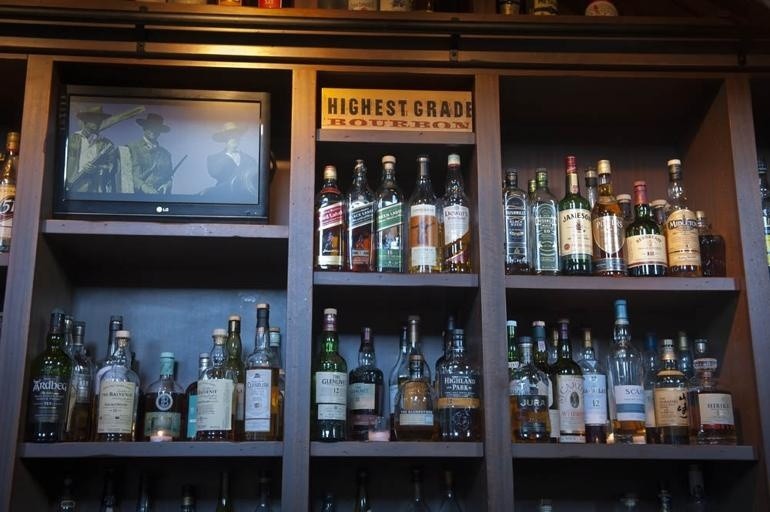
[51,84,271,225]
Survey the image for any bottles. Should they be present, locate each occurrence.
[26,302,284,441]
[316,152,475,272]
[311,306,483,442]
[504,299,742,445]
[0,126,21,253]
[504,154,728,276]
[49,465,716,510]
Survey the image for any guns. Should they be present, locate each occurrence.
[68,144,112,190]
[153,154,186,189]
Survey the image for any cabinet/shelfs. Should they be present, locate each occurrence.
[0,0,770,512]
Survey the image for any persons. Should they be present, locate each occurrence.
[205,122,257,202]
[64,105,116,196]
[119,113,173,193]
